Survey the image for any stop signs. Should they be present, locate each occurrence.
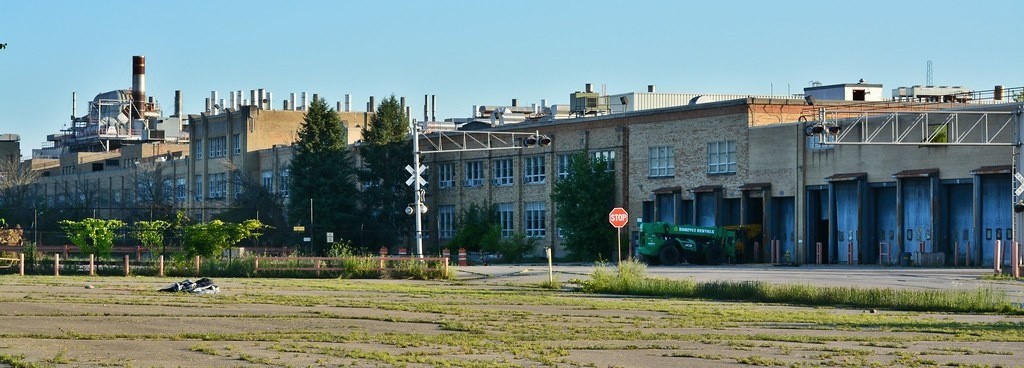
[608,208,629,228]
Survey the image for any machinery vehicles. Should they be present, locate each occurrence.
[637,222,762,265]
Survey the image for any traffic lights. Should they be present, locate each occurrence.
[829,126,841,135]
[538,136,551,147]
[524,137,536,146]
[812,126,824,135]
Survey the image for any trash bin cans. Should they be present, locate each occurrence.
[899,252,912,266]
